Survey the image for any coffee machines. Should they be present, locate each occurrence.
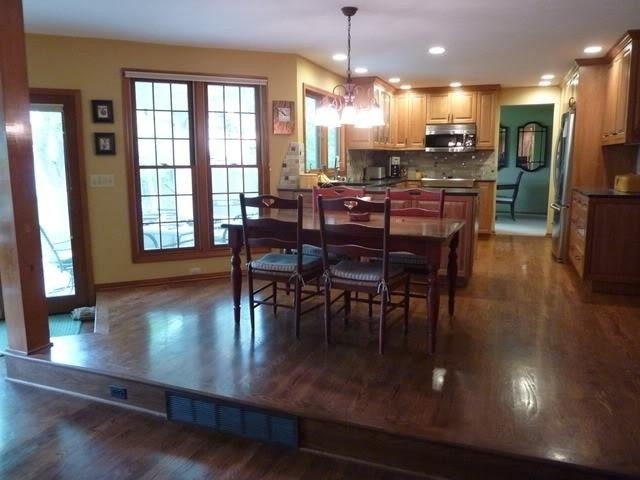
[387,155,401,178]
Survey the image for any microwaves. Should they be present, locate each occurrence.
[425,123,477,153]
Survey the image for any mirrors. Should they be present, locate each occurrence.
[516,121,548,172]
[498,124,508,169]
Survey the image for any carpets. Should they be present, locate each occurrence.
[0,312,84,337]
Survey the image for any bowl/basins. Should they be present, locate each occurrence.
[299,174,318,190]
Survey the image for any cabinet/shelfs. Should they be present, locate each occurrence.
[427,86,476,125]
[347,75,395,153]
[475,179,496,235]
[394,87,427,151]
[474,85,500,152]
[568,192,639,293]
[602,30,640,148]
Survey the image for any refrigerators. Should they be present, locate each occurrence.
[550,110,576,263]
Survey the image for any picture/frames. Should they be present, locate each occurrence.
[93,132,117,157]
[91,99,113,124]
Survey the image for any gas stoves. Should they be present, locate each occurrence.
[422,178,475,189]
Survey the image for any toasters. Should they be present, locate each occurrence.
[365,166,386,180]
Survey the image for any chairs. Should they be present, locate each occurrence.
[316,194,413,357]
[495,169,525,221]
[285,184,366,303]
[237,191,352,339]
[376,185,446,299]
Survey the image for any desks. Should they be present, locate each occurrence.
[220,208,467,358]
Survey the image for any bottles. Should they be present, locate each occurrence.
[439,172,447,179]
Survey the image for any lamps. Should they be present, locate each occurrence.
[303,7,387,129]
